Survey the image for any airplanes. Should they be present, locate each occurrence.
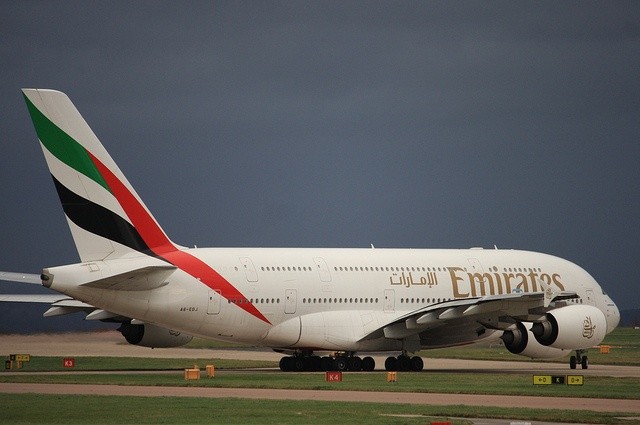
[0,87,621,370]
